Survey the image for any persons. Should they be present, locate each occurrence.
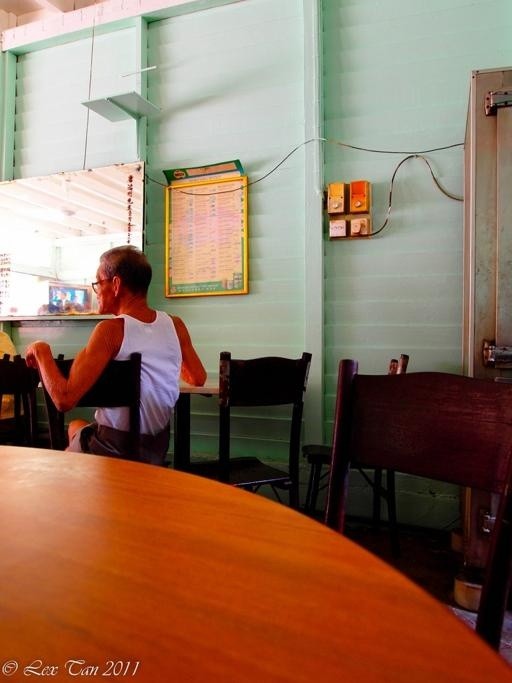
[56,291,84,311]
[24,243,208,468]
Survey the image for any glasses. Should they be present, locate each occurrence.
[92,279,110,293]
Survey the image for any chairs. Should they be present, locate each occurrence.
[326,359,510,654]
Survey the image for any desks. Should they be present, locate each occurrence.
[0,444,512,682]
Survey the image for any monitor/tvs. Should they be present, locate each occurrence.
[48,282,92,313]
